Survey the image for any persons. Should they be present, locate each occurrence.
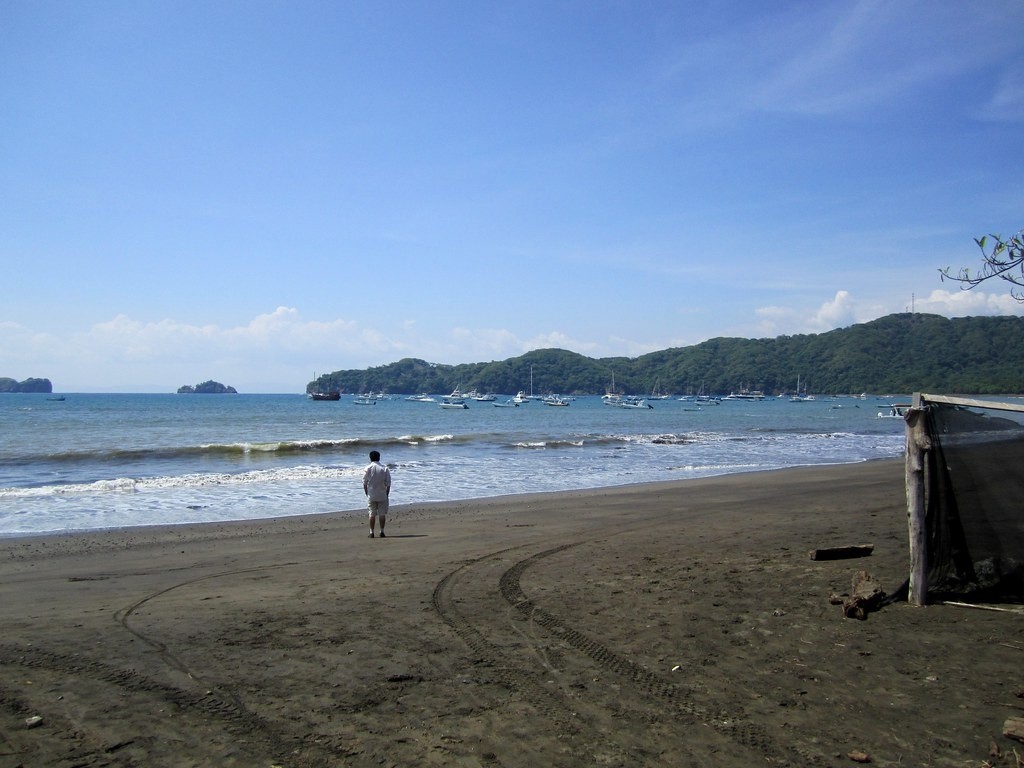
[363,451,391,538]
[890,408,902,416]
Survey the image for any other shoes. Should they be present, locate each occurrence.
[368,533,374,538]
[380,532,385,537]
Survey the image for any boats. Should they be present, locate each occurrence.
[307,364,914,419]
[45,395,66,401]
